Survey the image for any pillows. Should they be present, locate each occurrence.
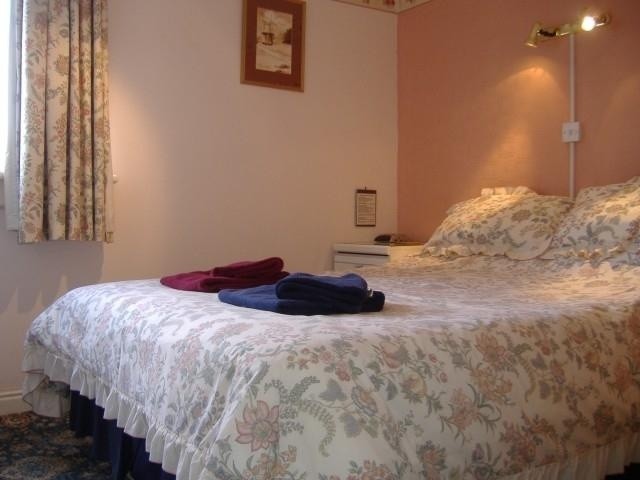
[539,176,639,265]
[423,186,570,261]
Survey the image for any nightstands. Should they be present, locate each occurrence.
[334,240,424,272]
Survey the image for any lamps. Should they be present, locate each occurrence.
[523,12,611,48]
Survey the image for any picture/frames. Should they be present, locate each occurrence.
[239,0,307,93]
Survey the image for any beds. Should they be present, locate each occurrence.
[22,252,640,480]
